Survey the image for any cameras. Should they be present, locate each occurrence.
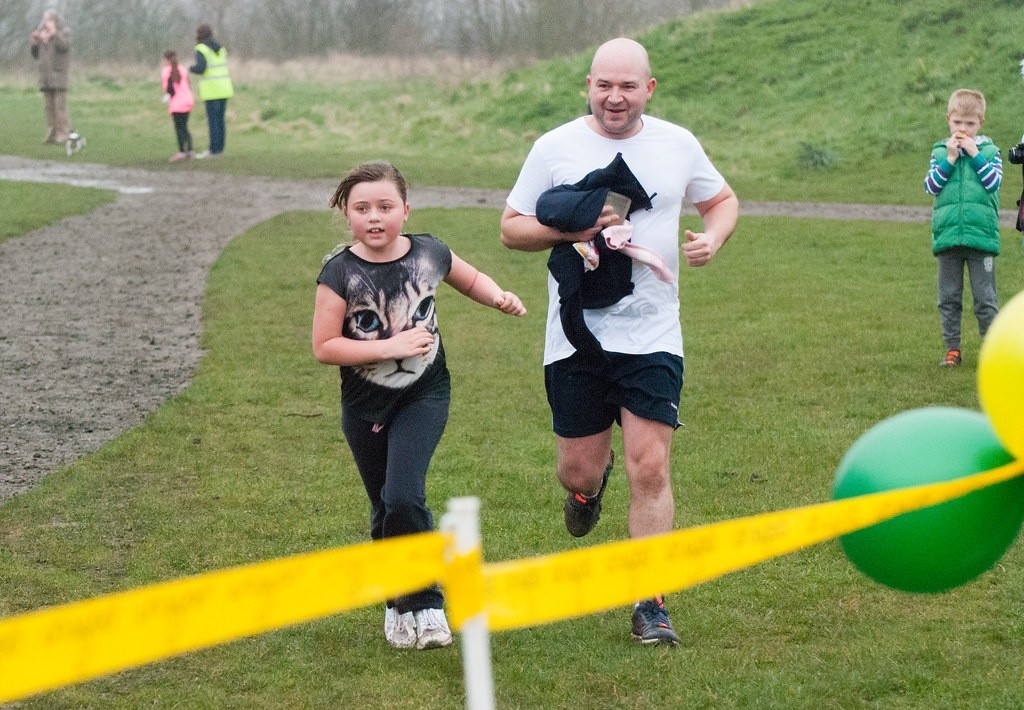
[1008,142,1024,164]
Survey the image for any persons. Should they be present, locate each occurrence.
[161,50,194,163]
[925,88,1002,368]
[501,37,739,644]
[188,24,232,160]
[313,162,526,648]
[32,9,71,142]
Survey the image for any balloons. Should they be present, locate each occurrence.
[832,406,1024,595]
[977,288,1023,461]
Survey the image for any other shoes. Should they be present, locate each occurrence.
[197,150,222,160]
[170,151,188,162]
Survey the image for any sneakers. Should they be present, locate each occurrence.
[945,349,961,364]
[565,448,615,540]
[631,595,679,646]
[385,605,418,651]
[414,608,452,650]
